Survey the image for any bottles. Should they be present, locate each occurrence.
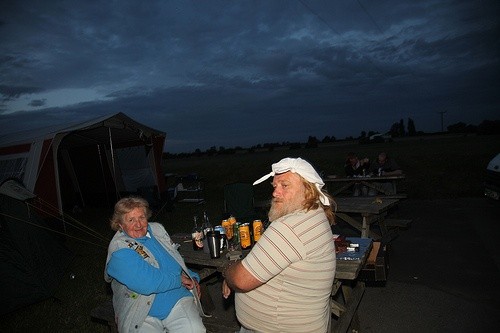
[201,211,210,239]
[192,216,203,251]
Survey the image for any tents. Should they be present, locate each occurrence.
[1,112,166,233]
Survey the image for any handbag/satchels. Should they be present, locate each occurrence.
[198,271,241,333]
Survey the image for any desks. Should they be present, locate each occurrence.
[324,175,406,197]
[170,232,373,333]
[253,191,400,252]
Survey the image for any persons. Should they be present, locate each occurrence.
[367,152,402,196]
[345,152,369,196]
[104,197,212,333]
[222,157,336,333]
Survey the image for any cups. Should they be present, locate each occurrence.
[207,231,226,259]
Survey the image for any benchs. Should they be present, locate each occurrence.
[384,194,409,198]
[365,242,381,264]
[91,300,241,333]
[352,217,412,228]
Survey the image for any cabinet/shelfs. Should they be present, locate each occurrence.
[174,189,206,204]
